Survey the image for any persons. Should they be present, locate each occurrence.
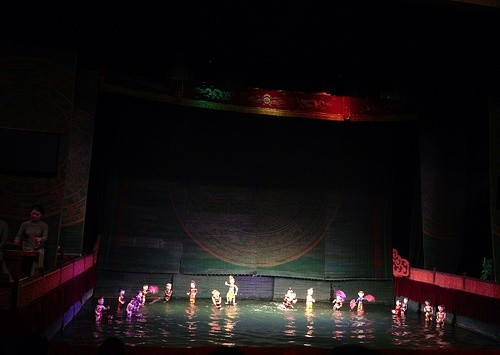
[0,220,9,246]
[211,289,222,306]
[332,294,343,310]
[126,284,154,319]
[306,288,315,309]
[13,208,49,273]
[94,296,110,327]
[187,280,198,304]
[225,275,238,305]
[435,305,447,327]
[164,283,173,301]
[354,290,369,315]
[117,289,126,316]
[423,300,434,322]
[284,288,297,308]
[393,297,409,316]
